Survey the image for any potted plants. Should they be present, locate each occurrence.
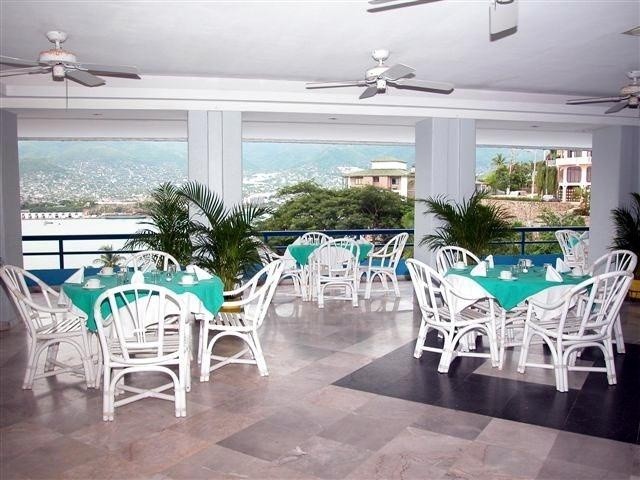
[605,192,640,299]
[176,180,269,313]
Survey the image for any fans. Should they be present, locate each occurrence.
[0,31,140,87]
[369,0,519,35]
[567,71,640,114]
[306,47,454,99]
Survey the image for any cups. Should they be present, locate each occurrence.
[510,264,522,274]
[180,275,194,282]
[85,278,101,288]
[484,259,489,271]
[185,265,195,272]
[151,270,161,284]
[100,266,114,274]
[453,262,464,268]
[522,259,531,265]
[116,271,126,286]
[501,271,512,278]
[165,264,177,282]
[570,267,583,275]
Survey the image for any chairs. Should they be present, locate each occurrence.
[405,228,638,392]
[250,232,409,308]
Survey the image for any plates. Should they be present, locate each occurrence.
[81,285,106,291]
[451,267,467,272]
[184,272,198,276]
[497,276,518,282]
[95,272,117,277]
[178,281,199,288]
[520,264,535,267]
[567,273,588,279]
